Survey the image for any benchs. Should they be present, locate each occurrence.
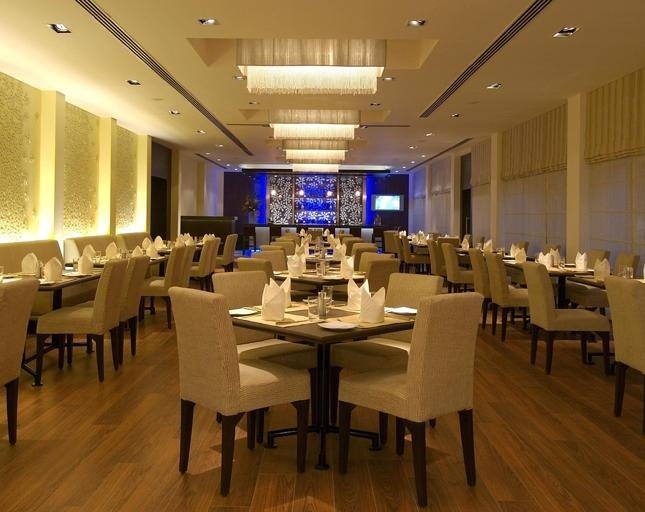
[116,232,153,252]
[63,234,122,268]
[0,240,65,274]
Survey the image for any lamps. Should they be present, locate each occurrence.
[266,110,360,142]
[292,158,340,172]
[285,141,346,163]
[236,41,390,95]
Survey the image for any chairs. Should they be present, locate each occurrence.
[190,239,214,292]
[358,252,395,272]
[185,245,197,288]
[210,269,268,305]
[232,233,239,271]
[402,236,431,274]
[216,234,234,272]
[250,250,287,271]
[275,233,301,246]
[510,240,529,257]
[261,245,285,250]
[484,251,529,342]
[270,241,295,255]
[354,246,378,270]
[523,261,611,376]
[167,286,312,495]
[235,257,275,284]
[34,257,135,388]
[386,273,446,311]
[613,252,640,278]
[426,240,447,277]
[438,237,459,248]
[393,233,404,273]
[353,242,376,248]
[64,255,150,364]
[536,243,561,264]
[369,259,400,296]
[0,277,41,445]
[461,234,472,248]
[343,236,363,256]
[585,249,611,269]
[603,274,645,436]
[336,291,484,510]
[441,242,474,293]
[210,236,221,292]
[139,245,186,329]
[468,248,521,330]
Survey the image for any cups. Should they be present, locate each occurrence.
[316,263,321,277]
[162,240,166,247]
[95,251,101,264]
[73,258,79,272]
[323,286,332,302]
[121,248,127,259]
[308,296,319,322]
[558,257,565,269]
[199,236,203,244]
[624,265,634,280]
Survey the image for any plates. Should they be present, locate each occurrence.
[61,272,93,277]
[316,320,360,330]
[2,277,23,284]
[229,307,258,315]
[302,298,335,306]
[37,278,56,284]
[385,306,418,315]
